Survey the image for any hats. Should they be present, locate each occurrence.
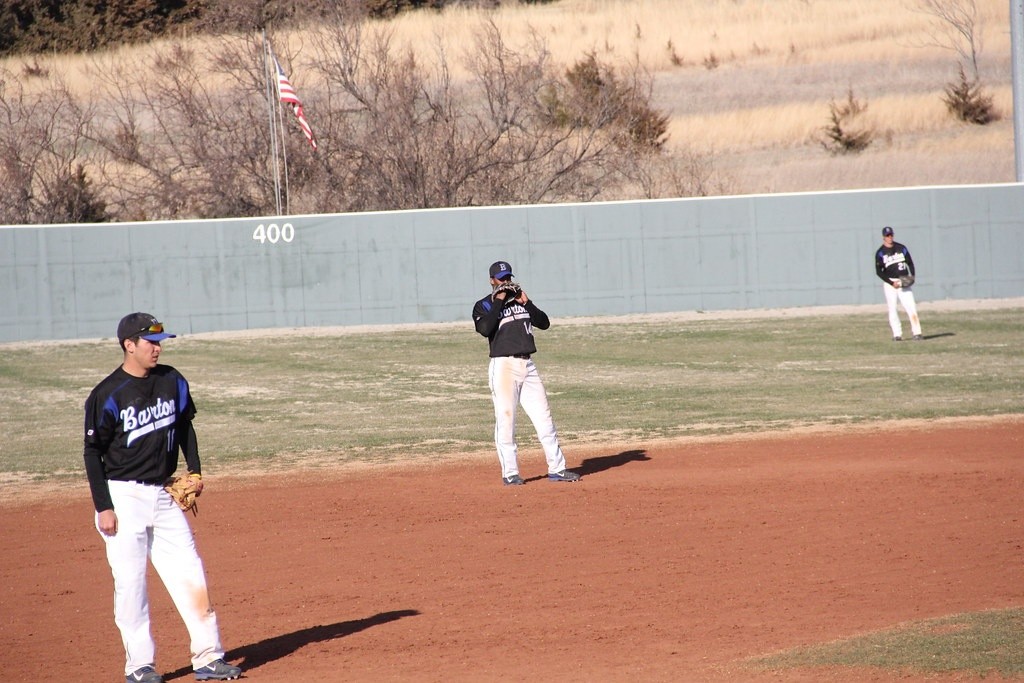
[489,261,515,279]
[882,227,894,235]
[117,312,177,342]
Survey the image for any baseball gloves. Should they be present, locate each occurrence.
[491,282,523,308]
[899,275,915,288]
[163,473,203,512]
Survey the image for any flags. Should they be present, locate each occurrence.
[272,54,317,152]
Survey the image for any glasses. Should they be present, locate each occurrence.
[123,322,164,338]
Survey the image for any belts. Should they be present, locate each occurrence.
[504,354,531,359]
[110,477,163,487]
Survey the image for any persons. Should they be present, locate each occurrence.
[471,262,580,485]
[83,311,242,683]
[875,227,923,340]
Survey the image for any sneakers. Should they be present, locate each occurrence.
[548,470,581,482]
[503,475,524,484]
[893,336,901,341]
[913,335,922,340]
[126,666,163,683]
[187,659,242,679]
